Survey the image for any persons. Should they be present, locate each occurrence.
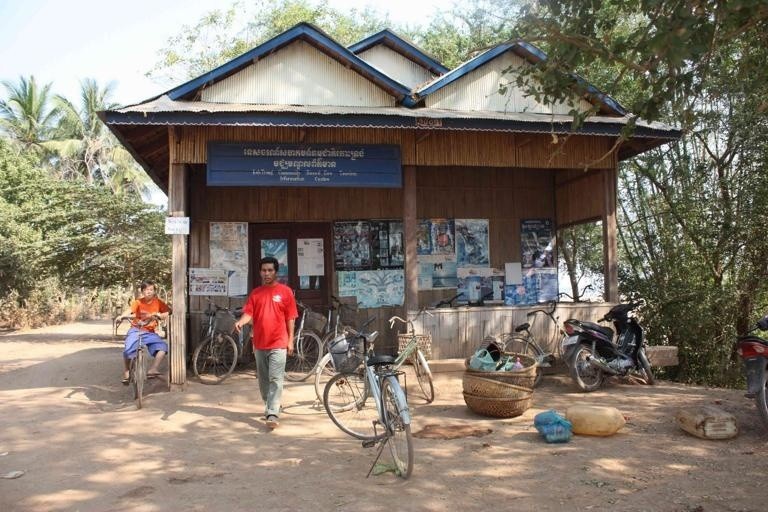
[115,280,172,385]
[233,256,299,429]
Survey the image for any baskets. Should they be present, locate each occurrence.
[328,338,363,374]
[396,330,432,364]
[303,311,327,334]
[462,351,536,418]
[212,312,238,337]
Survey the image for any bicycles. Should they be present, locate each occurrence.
[323,317,413,479]
[193,298,253,386]
[504,298,569,389]
[315,307,435,412]
[286,295,358,381]
[114,314,165,408]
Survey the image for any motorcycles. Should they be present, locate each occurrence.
[735,318,768,425]
[563,301,655,392]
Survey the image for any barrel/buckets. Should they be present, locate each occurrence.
[565,404,631,436]
[676,400,737,440]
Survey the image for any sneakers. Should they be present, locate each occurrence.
[265,416,279,429]
